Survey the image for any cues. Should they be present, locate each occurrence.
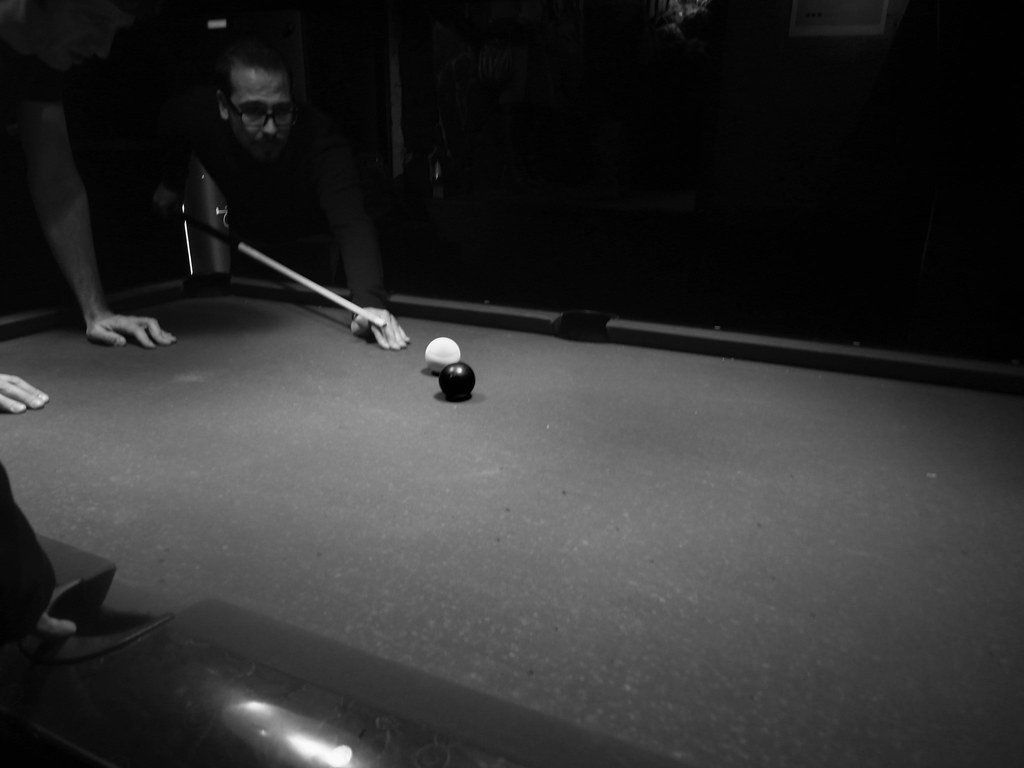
[179,209,388,329]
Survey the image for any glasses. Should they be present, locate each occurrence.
[224,101,297,126]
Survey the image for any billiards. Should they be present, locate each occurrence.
[425,336,476,399]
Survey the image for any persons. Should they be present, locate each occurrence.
[151,38,411,351]
[0,0,177,413]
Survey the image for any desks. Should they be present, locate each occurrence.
[0,269,1024,768]
